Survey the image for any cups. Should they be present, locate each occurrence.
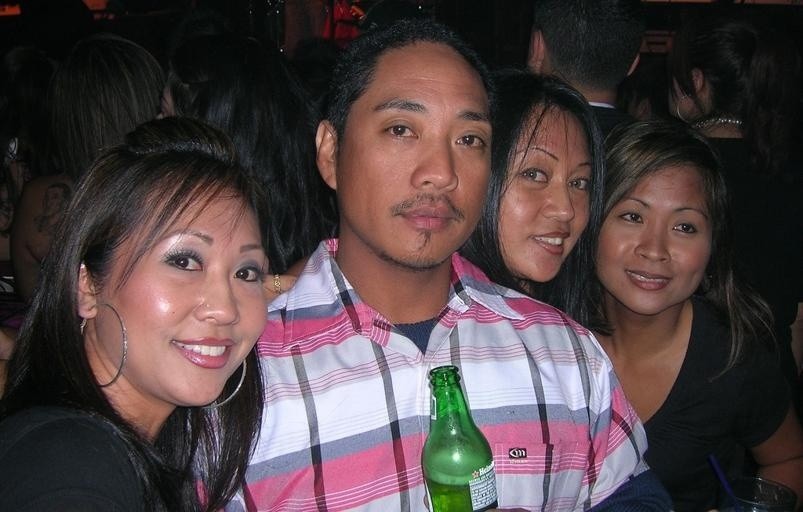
[719,475,798,512]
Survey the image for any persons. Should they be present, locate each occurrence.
[1,2,802,512]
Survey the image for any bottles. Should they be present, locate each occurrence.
[421,364,498,512]
